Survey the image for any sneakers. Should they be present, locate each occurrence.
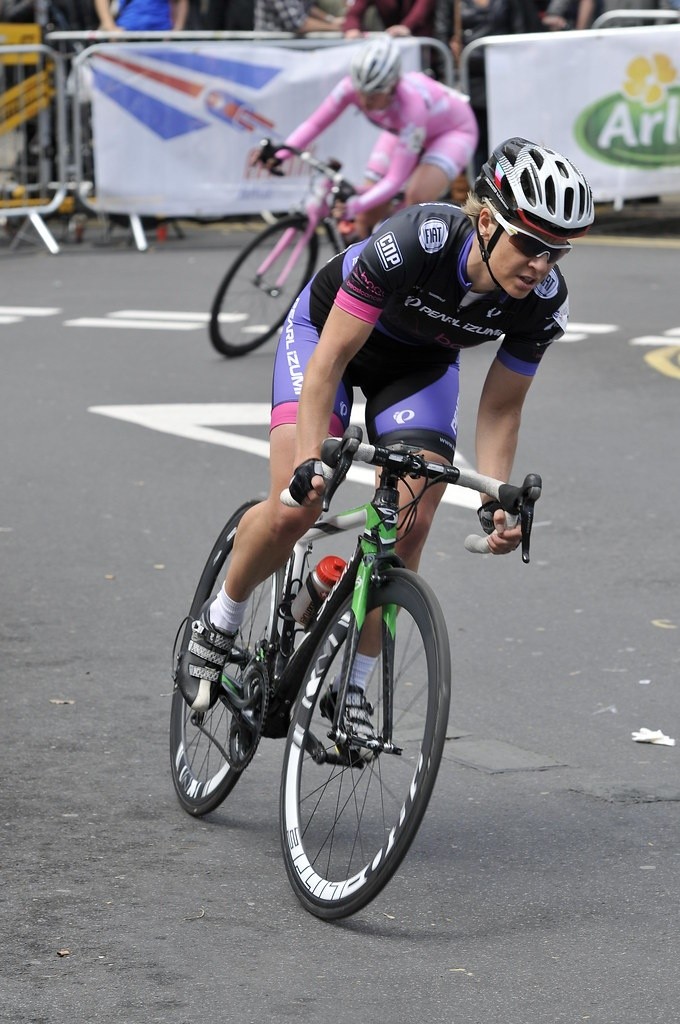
[176,596,239,711]
[320,683,376,760]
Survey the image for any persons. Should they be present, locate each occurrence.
[175,138,595,761]
[245,39,480,237]
[0,0,678,227]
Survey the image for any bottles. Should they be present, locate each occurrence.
[338,220,356,245]
[291,556,348,627]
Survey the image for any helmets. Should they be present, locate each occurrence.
[482,136,595,241]
[350,31,400,94]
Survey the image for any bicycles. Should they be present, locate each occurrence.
[205,133,375,361]
[168,417,544,925]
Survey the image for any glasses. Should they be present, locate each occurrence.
[480,197,573,264]
